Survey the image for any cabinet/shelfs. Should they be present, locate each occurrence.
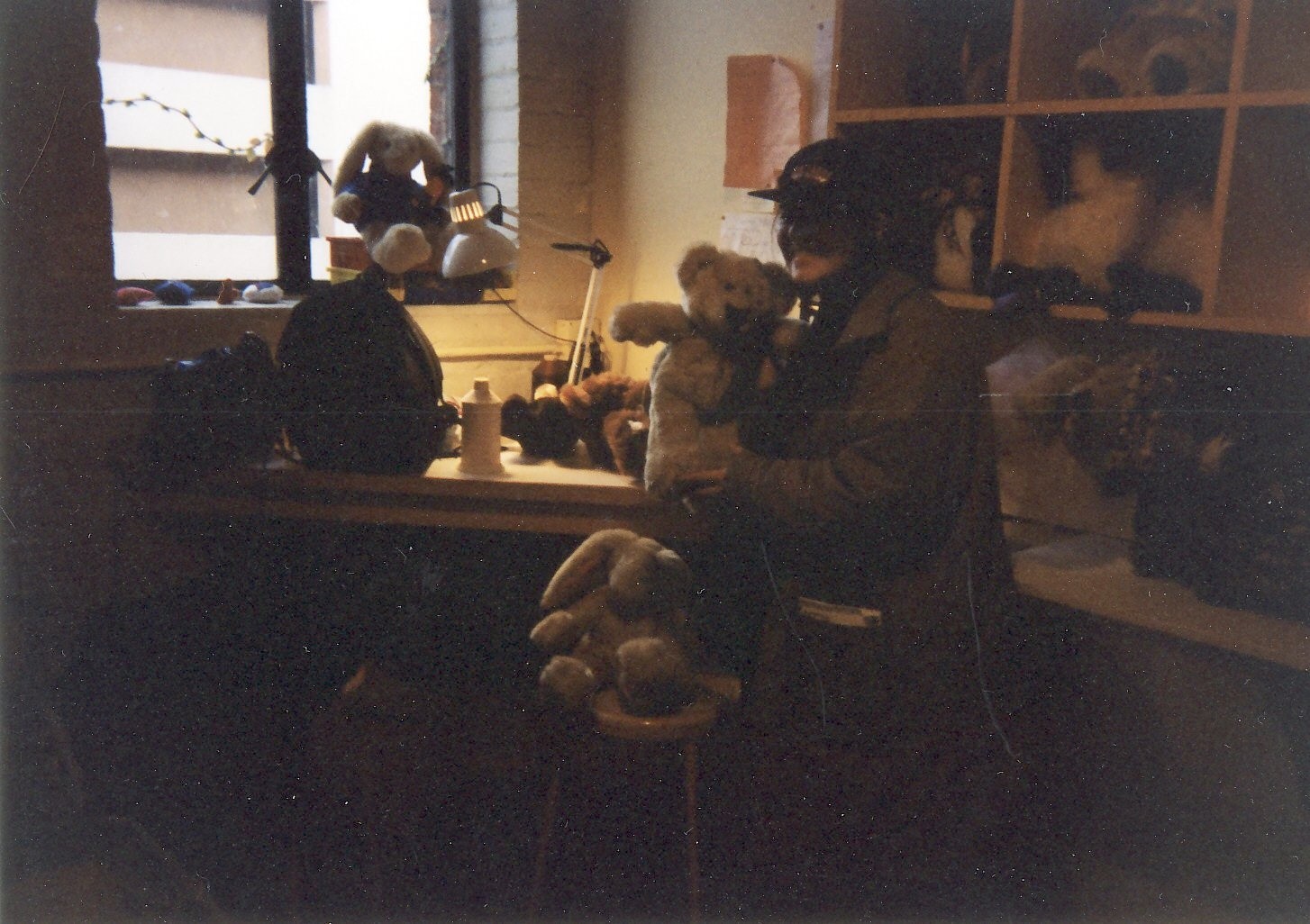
[824,0,1310,340]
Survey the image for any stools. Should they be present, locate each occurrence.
[529,682,723,924]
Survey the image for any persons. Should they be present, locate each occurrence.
[675,138,1013,587]
[1116,362,1310,620]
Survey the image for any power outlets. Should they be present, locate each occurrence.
[553,318,600,345]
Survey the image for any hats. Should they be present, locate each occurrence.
[747,138,897,207]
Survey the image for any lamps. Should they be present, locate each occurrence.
[442,188,610,389]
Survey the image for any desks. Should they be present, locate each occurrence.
[112,442,714,587]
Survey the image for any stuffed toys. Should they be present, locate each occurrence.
[893,1,1236,323]
[611,244,804,502]
[330,121,454,275]
[499,359,651,476]
[529,529,701,717]
